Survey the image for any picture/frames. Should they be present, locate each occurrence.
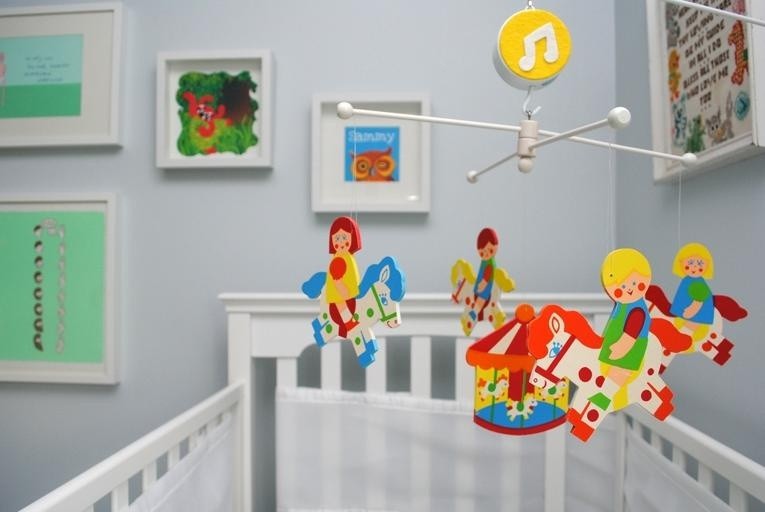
[644,0,765,185]
[0,0,126,148]
[1,193,122,387]
[310,92,432,216]
[155,51,278,169]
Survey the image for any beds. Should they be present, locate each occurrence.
[0,290,761,510]
